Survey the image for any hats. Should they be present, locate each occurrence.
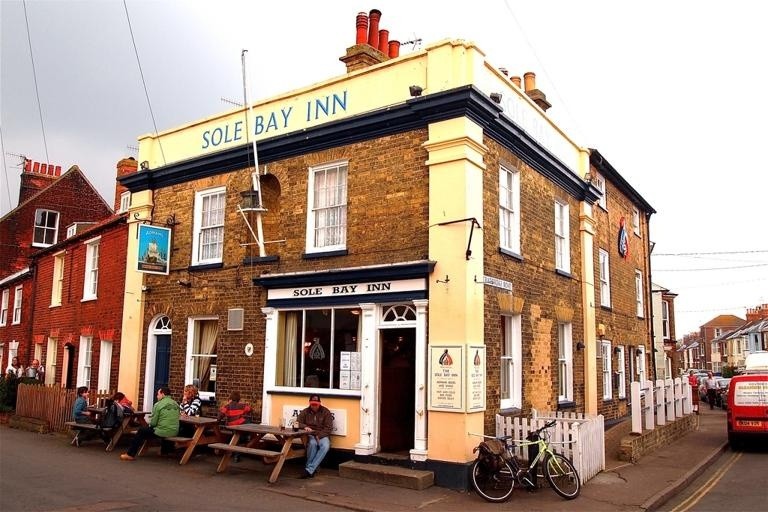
[309,395,320,404]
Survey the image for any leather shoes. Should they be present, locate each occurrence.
[301,470,316,478]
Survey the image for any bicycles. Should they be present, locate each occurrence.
[468,419,582,500]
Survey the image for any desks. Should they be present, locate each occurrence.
[65,407,321,483]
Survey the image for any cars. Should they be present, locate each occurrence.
[677,366,731,407]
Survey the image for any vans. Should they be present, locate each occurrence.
[723,369,768,452]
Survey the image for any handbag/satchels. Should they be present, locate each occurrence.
[478,440,506,473]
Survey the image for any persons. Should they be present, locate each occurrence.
[704,371,721,410]
[688,369,700,386]
[25,357,46,381]
[69,382,255,464]
[293,393,336,480]
[3,356,25,382]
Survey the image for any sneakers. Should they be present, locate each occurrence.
[234,457,240,463]
[104,439,112,451]
[121,454,134,459]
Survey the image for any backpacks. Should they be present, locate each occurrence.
[101,404,120,428]
